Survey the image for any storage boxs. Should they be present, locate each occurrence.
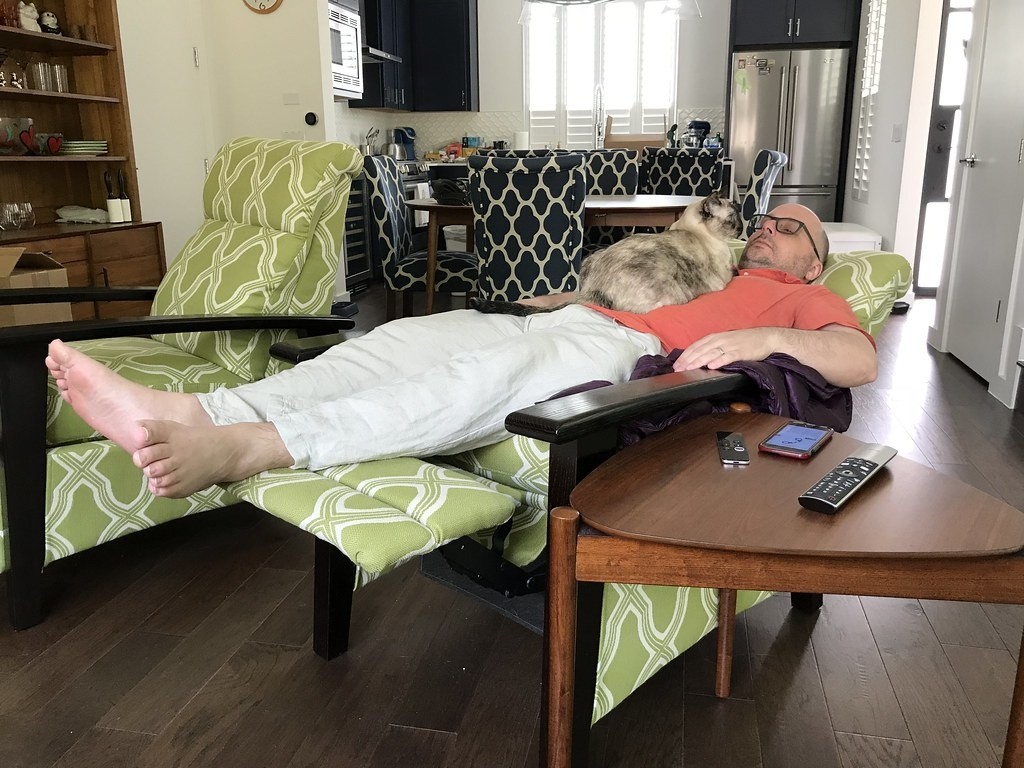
[603,114,668,149]
[0,244,75,328]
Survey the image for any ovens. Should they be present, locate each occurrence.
[395,161,431,242]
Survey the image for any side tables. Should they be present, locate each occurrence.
[545,410,1023,767]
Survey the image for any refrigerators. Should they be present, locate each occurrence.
[729,48,850,223]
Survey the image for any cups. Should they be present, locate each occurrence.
[51,64,70,92]
[32,62,52,91]
[387,129,396,143]
[360,145,374,156]
[0,202,36,231]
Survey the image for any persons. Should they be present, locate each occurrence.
[45,203,879,499]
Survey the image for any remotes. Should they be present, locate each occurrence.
[797,443,898,516]
[716,431,750,465]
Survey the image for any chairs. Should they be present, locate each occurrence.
[220,243,912,768]
[364,145,787,315]
[1,136,360,637]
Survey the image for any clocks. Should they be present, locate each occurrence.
[243,0,282,14]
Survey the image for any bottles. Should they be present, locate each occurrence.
[503,142,509,149]
[544,141,560,149]
[716,132,723,147]
[449,152,455,160]
[482,137,486,148]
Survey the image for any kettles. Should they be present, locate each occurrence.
[395,127,417,160]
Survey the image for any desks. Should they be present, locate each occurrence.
[405,190,706,314]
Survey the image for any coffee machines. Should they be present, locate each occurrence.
[681,120,711,148]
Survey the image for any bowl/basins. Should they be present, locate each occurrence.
[31,132,63,155]
[380,143,407,160]
[0,117,33,155]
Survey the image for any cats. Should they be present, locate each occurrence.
[467,190,743,317]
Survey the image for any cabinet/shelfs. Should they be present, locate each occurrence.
[414,0,479,112]
[0,1,169,320]
[732,0,862,47]
[348,0,410,113]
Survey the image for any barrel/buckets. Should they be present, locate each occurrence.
[442,225,466,296]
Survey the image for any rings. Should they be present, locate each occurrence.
[717,347,725,354]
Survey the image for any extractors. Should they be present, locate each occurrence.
[358,0,402,63]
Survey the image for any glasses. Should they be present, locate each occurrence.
[747,214,821,260]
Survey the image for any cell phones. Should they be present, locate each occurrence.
[758,419,834,460]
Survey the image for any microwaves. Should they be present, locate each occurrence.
[328,2,364,102]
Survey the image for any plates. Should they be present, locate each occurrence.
[58,141,108,157]
